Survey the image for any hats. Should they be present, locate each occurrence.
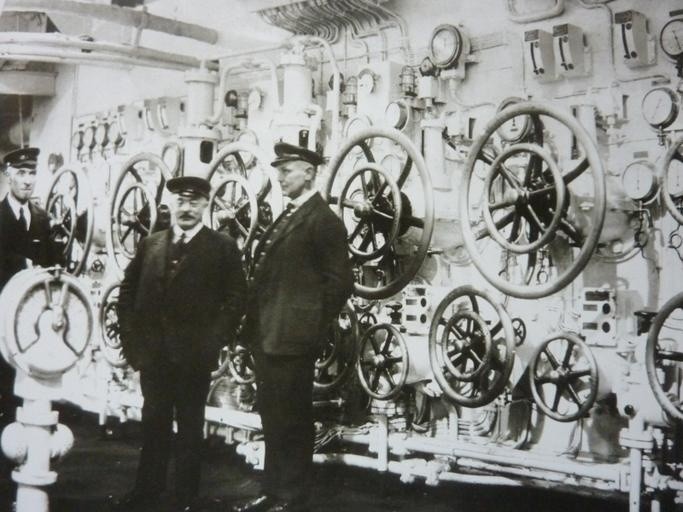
[271,142,323,166]
[167,176,210,200]
[4,148,39,168]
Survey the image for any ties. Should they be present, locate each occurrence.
[249,203,294,281]
[170,234,186,273]
[19,208,27,232]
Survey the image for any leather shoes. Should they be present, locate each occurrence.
[178,497,202,511]
[232,493,306,512]
[111,488,161,506]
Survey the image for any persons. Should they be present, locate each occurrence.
[0,146,57,460]
[234,141,355,512]
[112,176,246,511]
